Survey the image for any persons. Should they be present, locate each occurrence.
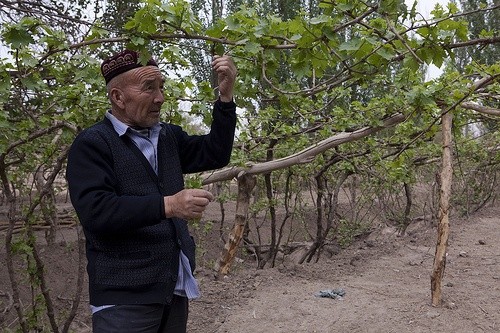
[66,53,236,333]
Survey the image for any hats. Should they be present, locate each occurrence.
[101,50,158,85]
